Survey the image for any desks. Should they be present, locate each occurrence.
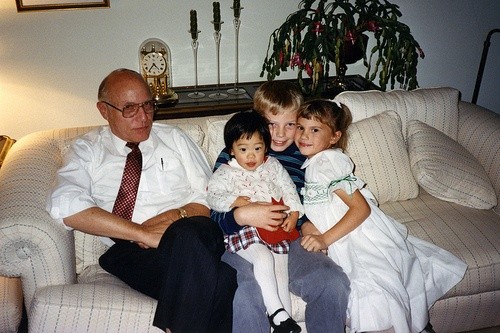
[153,74,386,122]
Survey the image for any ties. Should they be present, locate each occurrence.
[109,142,142,242]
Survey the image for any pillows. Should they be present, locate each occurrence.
[57,124,204,273]
[340,109,420,204]
[405,120,498,211]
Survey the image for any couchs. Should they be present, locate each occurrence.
[0,86,500,333]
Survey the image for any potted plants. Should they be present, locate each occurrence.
[259,0,426,93]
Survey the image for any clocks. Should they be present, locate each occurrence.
[139,42,172,101]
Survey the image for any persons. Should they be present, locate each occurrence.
[47,67,239,333]
[204,112,303,333]
[288,98,442,333]
[209,80,351,333]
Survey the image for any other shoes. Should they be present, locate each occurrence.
[268,308,302,333]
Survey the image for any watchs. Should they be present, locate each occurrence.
[175,207,189,219]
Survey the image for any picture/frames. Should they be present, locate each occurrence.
[15,0,110,13]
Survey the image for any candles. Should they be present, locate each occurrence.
[189,9,198,40]
[212,1,221,30]
[232,0,242,19]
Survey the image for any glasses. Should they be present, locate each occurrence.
[103,97,157,118]
